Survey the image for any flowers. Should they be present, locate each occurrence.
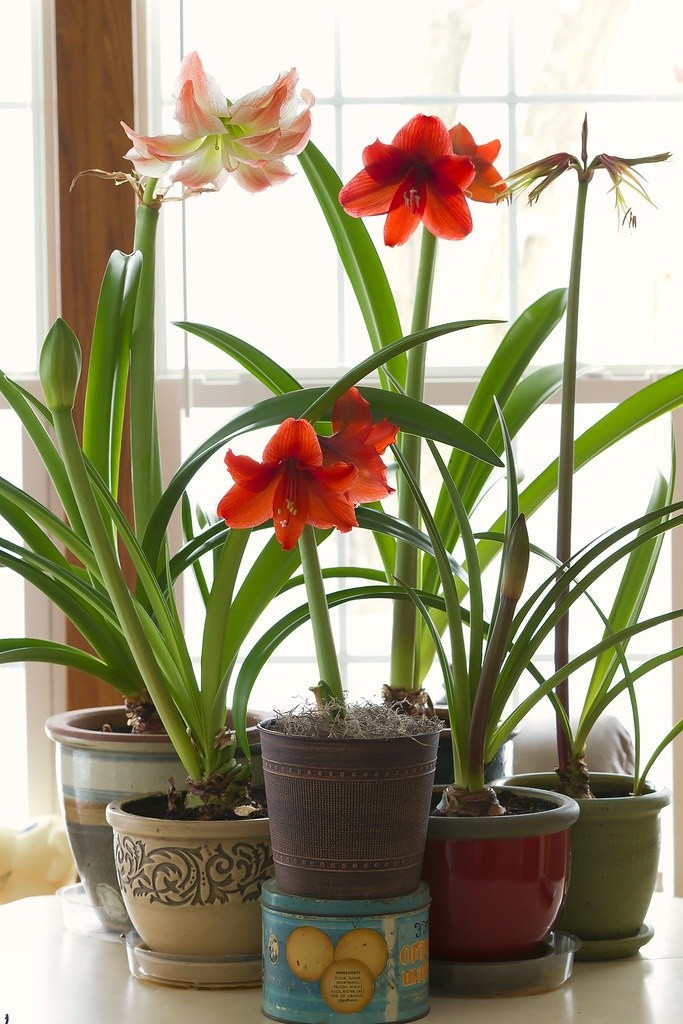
[217,382,400,722]
[0,47,683,817]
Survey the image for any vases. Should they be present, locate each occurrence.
[421,780,583,998]
[487,770,673,962]
[103,790,272,989]
[422,703,523,785]
[44,703,274,943]
[255,718,445,900]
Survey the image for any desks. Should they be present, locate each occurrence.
[0,893,683,1024]
